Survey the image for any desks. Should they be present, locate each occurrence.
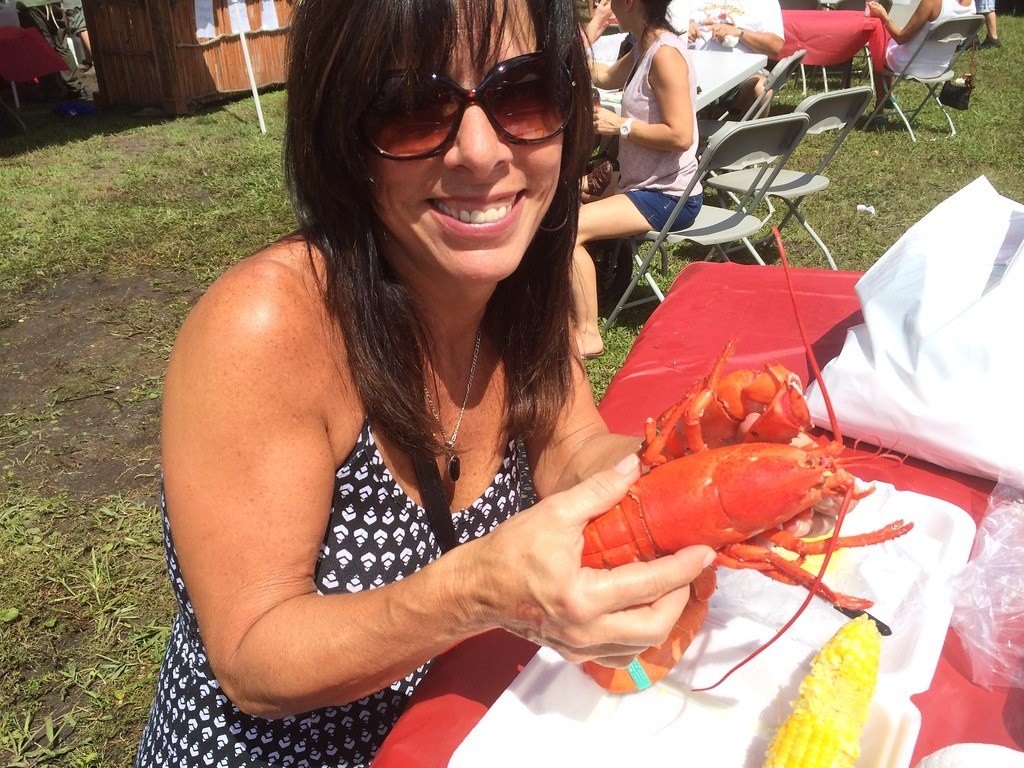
[371,261,1024,768]
[586,28,769,117]
[0,25,70,108]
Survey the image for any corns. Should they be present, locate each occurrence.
[761,612,883,768]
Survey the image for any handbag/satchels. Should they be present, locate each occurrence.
[939,80,972,110]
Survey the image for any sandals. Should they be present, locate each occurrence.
[587,161,613,196]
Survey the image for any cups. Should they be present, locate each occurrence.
[864,0,878,16]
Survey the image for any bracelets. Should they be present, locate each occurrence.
[738,27,744,38]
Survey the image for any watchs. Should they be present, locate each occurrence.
[619,118,633,140]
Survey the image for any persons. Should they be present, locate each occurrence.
[956,0,1001,52]
[132,0,860,768]
[867,0,977,125]
[15,0,93,101]
[568,0,703,356]
[665,0,786,122]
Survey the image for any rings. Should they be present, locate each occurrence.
[716,33,717,37]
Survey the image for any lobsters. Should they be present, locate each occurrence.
[581,222,917,694]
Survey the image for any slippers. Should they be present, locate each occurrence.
[581,349,604,357]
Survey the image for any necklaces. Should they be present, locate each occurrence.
[422,326,482,484]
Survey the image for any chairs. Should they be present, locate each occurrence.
[602,13,984,332]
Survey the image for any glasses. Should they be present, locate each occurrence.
[352,52,577,161]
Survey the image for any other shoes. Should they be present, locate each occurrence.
[884,96,898,108]
[863,116,889,129]
[954,35,982,52]
[980,33,1001,49]
